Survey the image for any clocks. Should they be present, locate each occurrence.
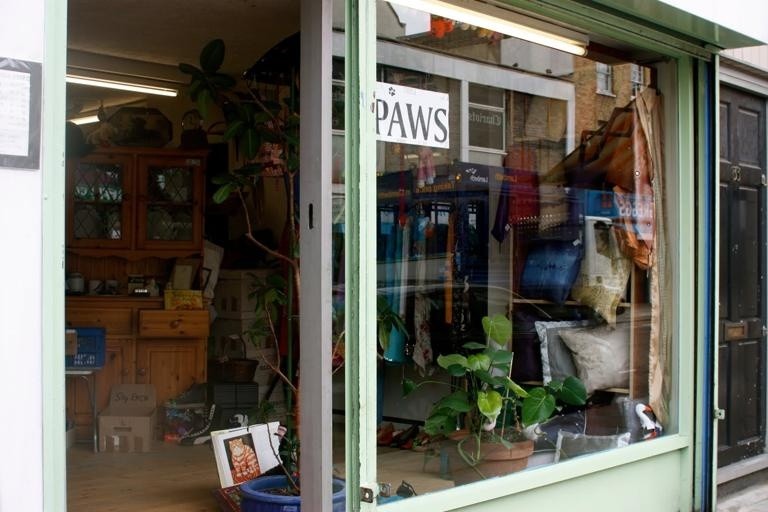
[181,110,203,133]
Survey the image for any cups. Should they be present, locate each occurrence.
[105,279,123,294]
[70,273,83,292]
[74,185,121,200]
[88,279,103,293]
[127,277,145,297]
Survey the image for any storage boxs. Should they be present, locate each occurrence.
[211,266,271,319]
[98,383,157,452]
[211,317,272,360]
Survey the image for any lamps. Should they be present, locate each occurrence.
[66,67,178,97]
[382,0,590,56]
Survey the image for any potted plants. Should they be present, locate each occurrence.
[400,312,586,487]
[179,39,409,512]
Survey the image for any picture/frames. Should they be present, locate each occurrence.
[332,195,345,225]
[331,79,345,133]
[332,132,345,194]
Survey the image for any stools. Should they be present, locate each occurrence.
[65,370,97,452]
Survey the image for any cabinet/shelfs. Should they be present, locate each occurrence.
[65,146,208,413]
[507,218,650,456]
[211,381,262,431]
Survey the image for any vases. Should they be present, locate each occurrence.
[210,357,259,384]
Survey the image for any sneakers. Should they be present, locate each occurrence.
[161,382,205,409]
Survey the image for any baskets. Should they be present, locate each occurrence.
[213,331,259,383]
[66,327,105,370]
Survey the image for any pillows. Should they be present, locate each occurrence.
[558,324,629,399]
[510,309,587,382]
[519,223,585,307]
[535,321,598,386]
[571,230,632,330]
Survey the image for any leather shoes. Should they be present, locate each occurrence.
[377,423,419,449]
